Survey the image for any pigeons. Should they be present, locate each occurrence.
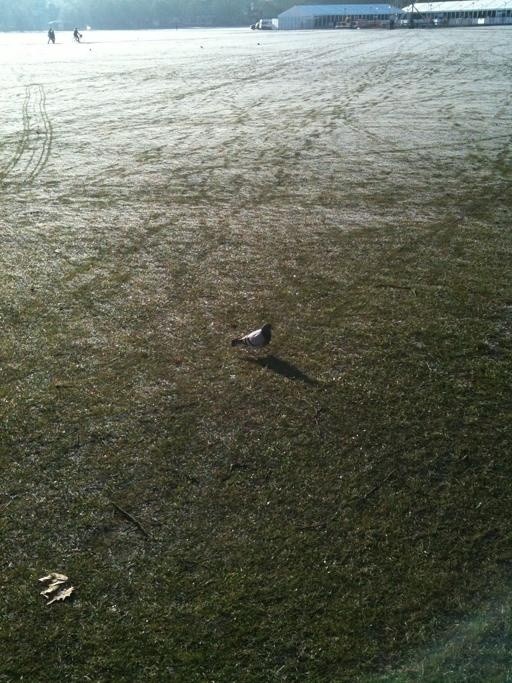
[230,324,273,353]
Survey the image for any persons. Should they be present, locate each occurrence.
[48,28,54,43]
[73,28,82,43]
